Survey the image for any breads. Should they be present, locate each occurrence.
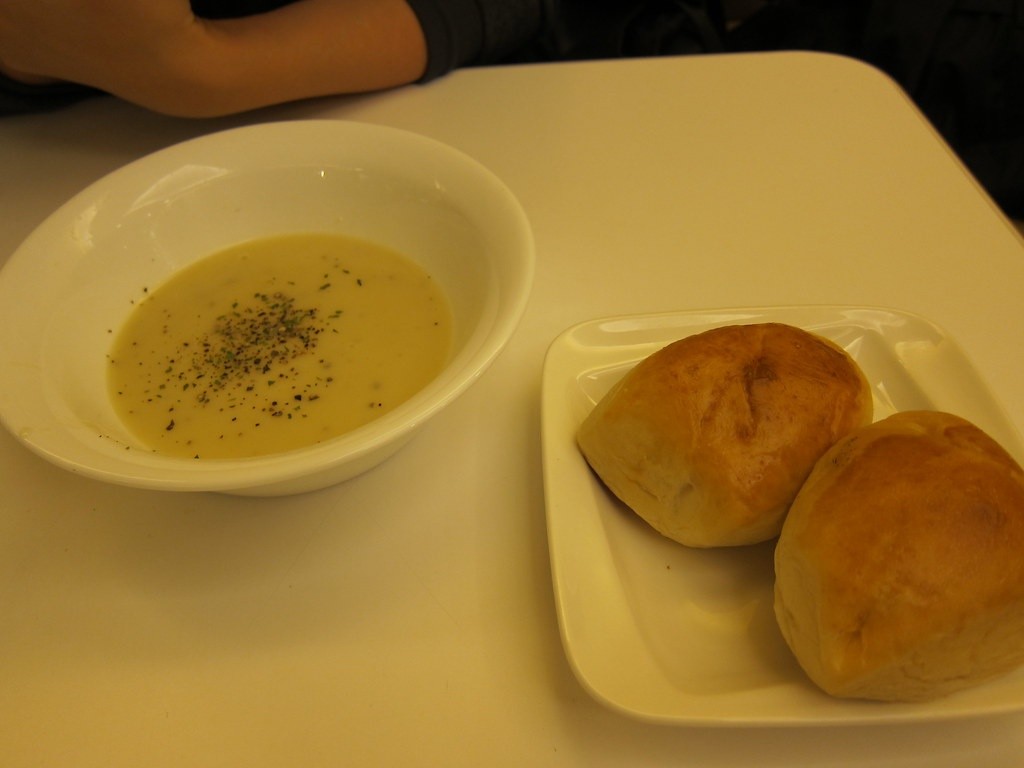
[773,410,1024,703]
[574,324,874,550]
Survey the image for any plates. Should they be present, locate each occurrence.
[541,306,1024,726]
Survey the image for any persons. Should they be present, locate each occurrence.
[0,0,604,121]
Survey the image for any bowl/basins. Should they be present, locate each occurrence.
[0,121,535,497]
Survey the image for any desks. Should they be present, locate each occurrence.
[0,52,1024,768]
